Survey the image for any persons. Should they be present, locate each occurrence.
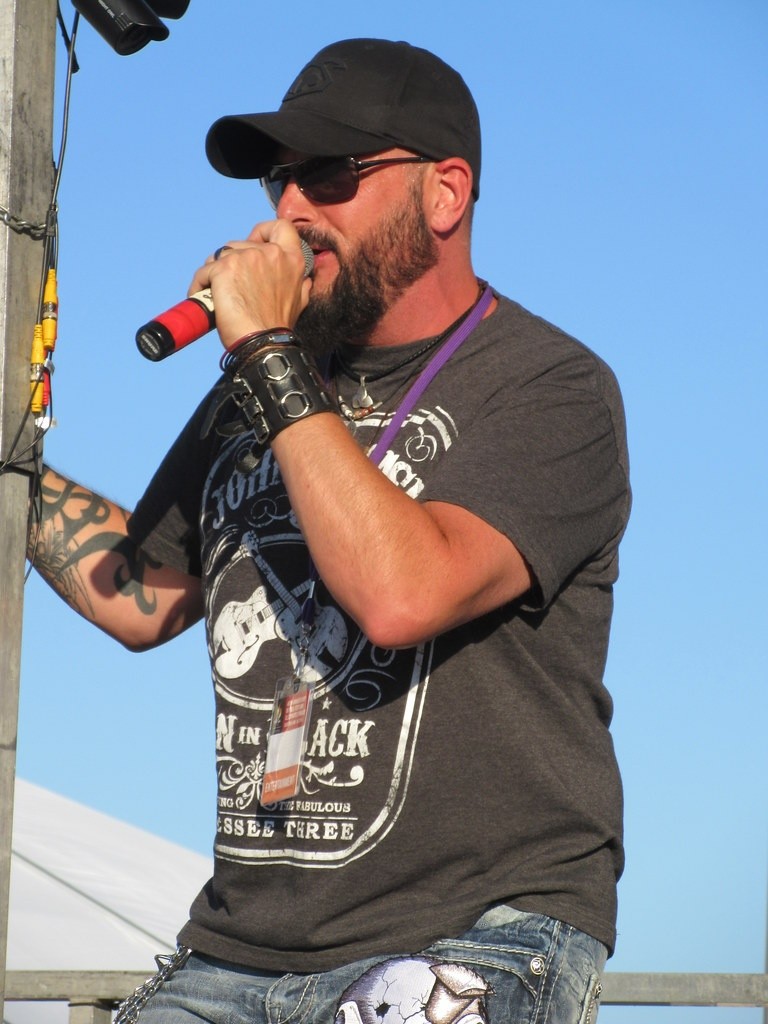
[24,38,632,1023]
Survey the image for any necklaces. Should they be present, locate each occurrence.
[326,282,482,454]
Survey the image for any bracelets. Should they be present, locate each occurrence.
[200,348,332,472]
[219,325,300,384]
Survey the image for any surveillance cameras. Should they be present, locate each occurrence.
[71,0,169,56]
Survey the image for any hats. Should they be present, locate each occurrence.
[203,35,482,198]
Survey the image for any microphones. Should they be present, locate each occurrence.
[135,238,315,362]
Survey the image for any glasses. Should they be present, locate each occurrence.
[259,147,435,209]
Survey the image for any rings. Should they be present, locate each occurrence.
[215,246,233,260]
